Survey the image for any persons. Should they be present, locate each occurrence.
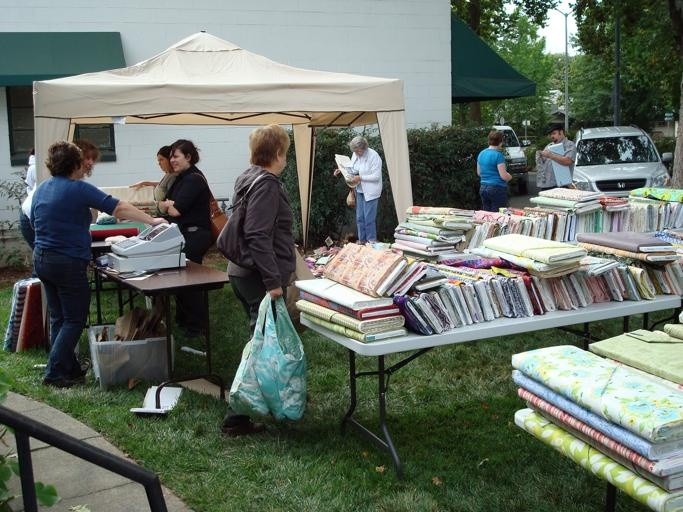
[221,124,296,434]
[129,146,176,202]
[476,132,512,212]
[25,148,36,193]
[333,136,383,245]
[541,123,577,190]
[158,139,216,332]
[19,140,99,250]
[30,142,169,389]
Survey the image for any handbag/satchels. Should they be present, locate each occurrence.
[216,172,276,270]
[229,290,306,423]
[346,188,355,208]
[190,172,229,240]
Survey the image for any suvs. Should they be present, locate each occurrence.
[571,123,672,199]
[474,125,528,194]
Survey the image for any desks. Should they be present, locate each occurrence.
[514,406,683,511]
[88,258,232,379]
[298,296,682,478]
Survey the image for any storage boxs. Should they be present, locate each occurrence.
[85,324,170,390]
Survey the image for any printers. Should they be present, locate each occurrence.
[106,222,187,272]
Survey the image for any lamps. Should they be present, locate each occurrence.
[111,116,127,126]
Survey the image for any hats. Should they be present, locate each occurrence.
[546,123,561,133]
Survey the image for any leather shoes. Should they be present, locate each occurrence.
[220,419,265,434]
